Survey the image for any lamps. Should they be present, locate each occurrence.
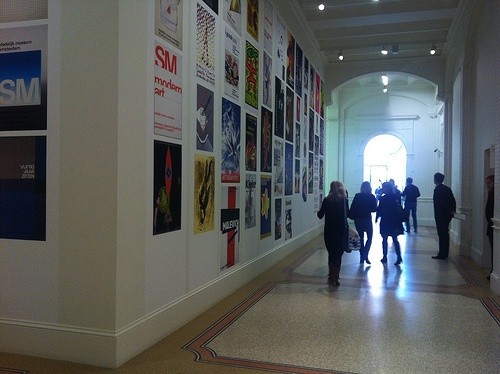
[429,42,437,56]
[338,50,344,61]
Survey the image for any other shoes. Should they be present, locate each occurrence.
[432,255,446,259]
[381,257,387,262]
[394,257,402,265]
[365,257,371,264]
[360,259,364,263]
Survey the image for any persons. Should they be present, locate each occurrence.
[401,178,420,232]
[349,182,377,264]
[376,179,405,231]
[431,173,456,259]
[375,182,404,265]
[317,181,349,287]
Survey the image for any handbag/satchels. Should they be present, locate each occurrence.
[345,228,361,253]
